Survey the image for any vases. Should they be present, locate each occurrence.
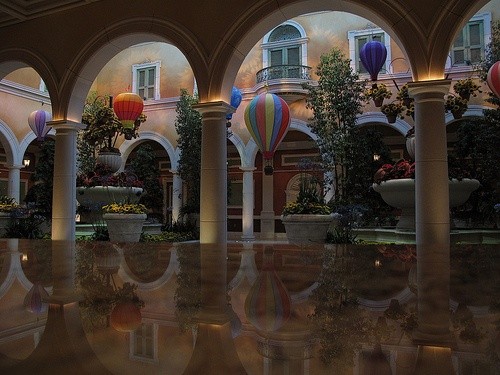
[0,212,14,238]
[102,214,147,242]
[372,178,481,230]
[282,213,338,247]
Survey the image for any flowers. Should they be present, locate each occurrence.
[98,197,149,215]
[0,195,20,212]
[281,158,332,216]
[373,154,475,185]
